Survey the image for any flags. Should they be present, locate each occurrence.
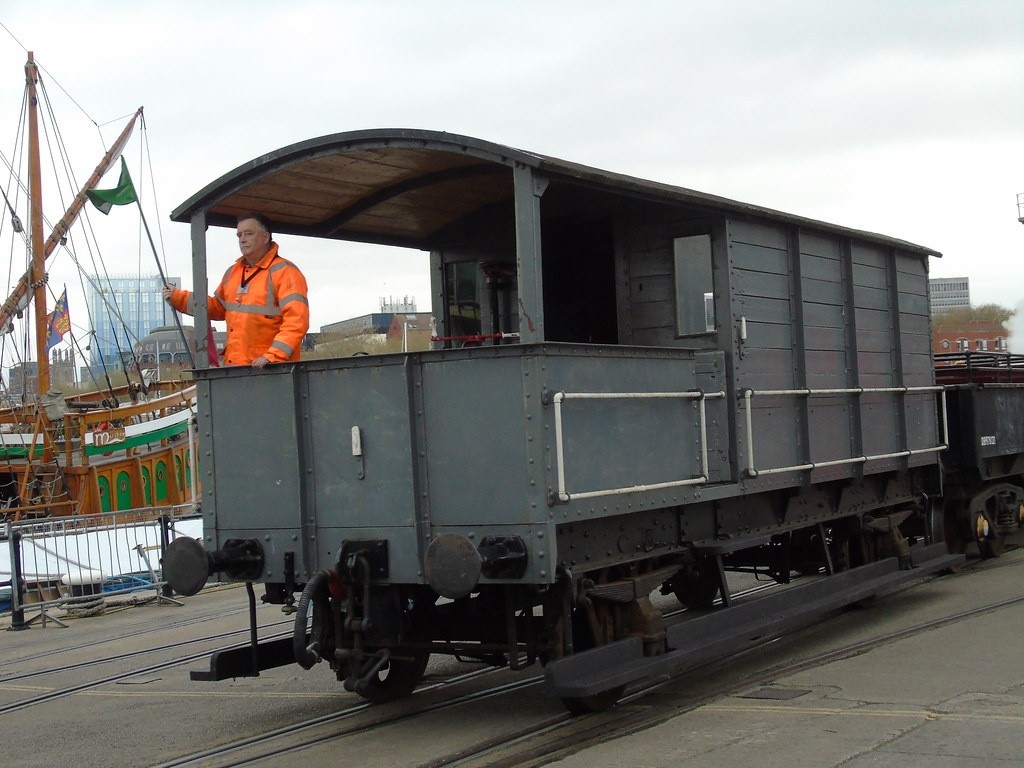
[45,289,70,356]
[83,160,137,215]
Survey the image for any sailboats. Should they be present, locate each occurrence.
[0,19,204,537]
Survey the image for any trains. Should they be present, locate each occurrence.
[168,125,1024,717]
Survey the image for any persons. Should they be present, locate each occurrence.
[160,213,310,371]
